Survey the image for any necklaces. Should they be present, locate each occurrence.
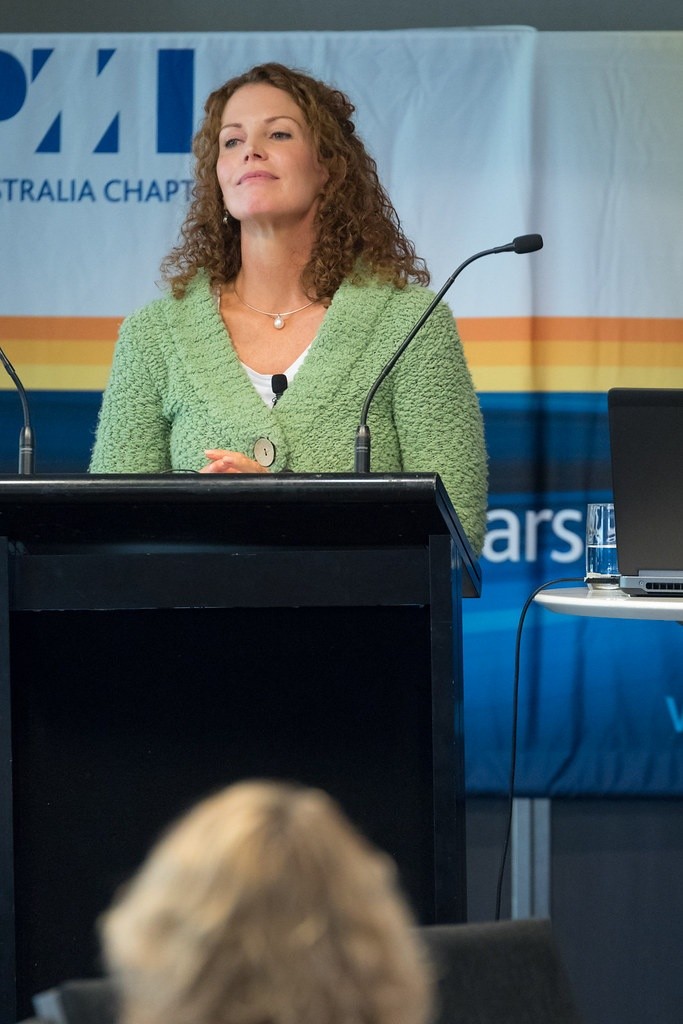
[233,280,319,330]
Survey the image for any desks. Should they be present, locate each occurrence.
[532,588,683,622]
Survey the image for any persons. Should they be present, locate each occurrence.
[100,784,432,1024]
[89,62,487,556]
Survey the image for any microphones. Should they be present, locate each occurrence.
[271,374,288,395]
[355,234,544,474]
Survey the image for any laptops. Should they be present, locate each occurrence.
[608,389,683,598]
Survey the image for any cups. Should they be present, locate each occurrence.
[585,502,623,589]
